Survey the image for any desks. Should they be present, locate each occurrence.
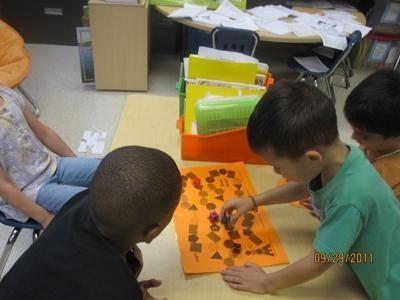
[108,96,368,300]
[155,1,367,62]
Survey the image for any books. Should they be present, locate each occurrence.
[167,6,229,28]
[246,4,301,20]
[183,46,269,135]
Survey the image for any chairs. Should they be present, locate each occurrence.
[210,27,260,58]
[0,214,44,278]
[310,42,353,90]
[287,30,361,100]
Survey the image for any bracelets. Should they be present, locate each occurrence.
[249,195,258,212]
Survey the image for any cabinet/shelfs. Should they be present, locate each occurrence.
[88,0,148,92]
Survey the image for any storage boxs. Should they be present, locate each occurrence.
[177,114,269,165]
[175,78,185,116]
[180,61,275,88]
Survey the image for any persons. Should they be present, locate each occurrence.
[304,67,400,215]
[1,85,101,229]
[0,145,182,300]
[220,78,400,300]
